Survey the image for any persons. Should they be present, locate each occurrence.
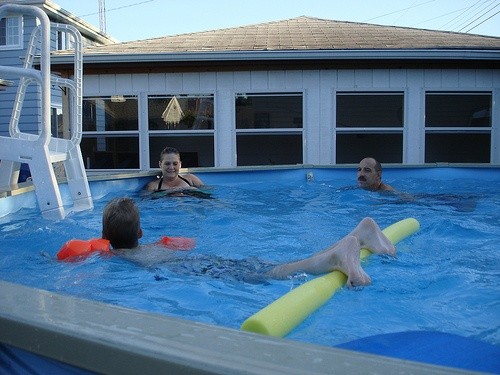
[145,146,204,190]
[356,155,397,191]
[101,198,395,290]
